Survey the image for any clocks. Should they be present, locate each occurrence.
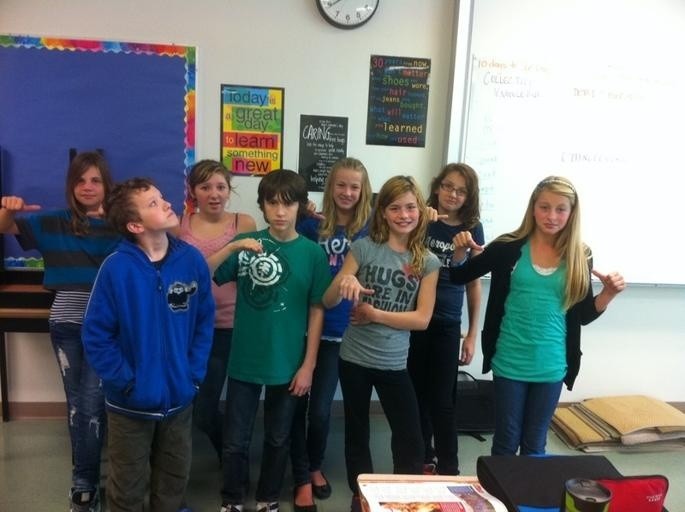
[314,0,379,30]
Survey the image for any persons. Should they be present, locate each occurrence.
[0,147,126,512]
[407,162,486,476]
[205,169,332,512]
[79,177,217,433]
[320,176,443,512]
[170,159,256,467]
[448,176,624,455]
[301,156,377,500]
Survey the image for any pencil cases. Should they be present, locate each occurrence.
[592,476,668,512]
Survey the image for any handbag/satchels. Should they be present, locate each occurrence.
[454,370,499,435]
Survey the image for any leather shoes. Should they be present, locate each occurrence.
[312,468,332,499]
[291,486,317,510]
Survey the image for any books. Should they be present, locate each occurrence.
[551,394,685,452]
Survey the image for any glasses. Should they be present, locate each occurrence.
[438,182,470,197]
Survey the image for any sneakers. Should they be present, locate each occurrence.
[258,502,276,510]
[221,504,241,510]
[68,479,102,512]
